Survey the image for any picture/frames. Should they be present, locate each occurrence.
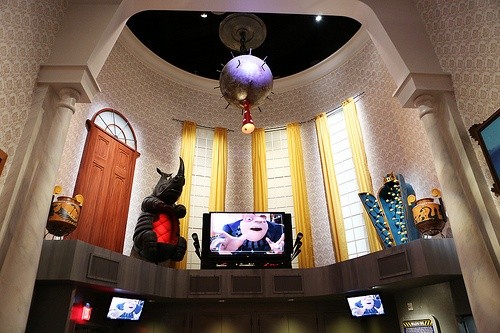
[468,108,500,197]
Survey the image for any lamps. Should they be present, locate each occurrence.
[242,100,256,135]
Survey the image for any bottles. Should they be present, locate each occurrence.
[46,196,80,236]
[411,198,444,234]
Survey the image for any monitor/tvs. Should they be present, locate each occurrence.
[346,294,385,319]
[201,211,294,269]
[106,296,144,320]
